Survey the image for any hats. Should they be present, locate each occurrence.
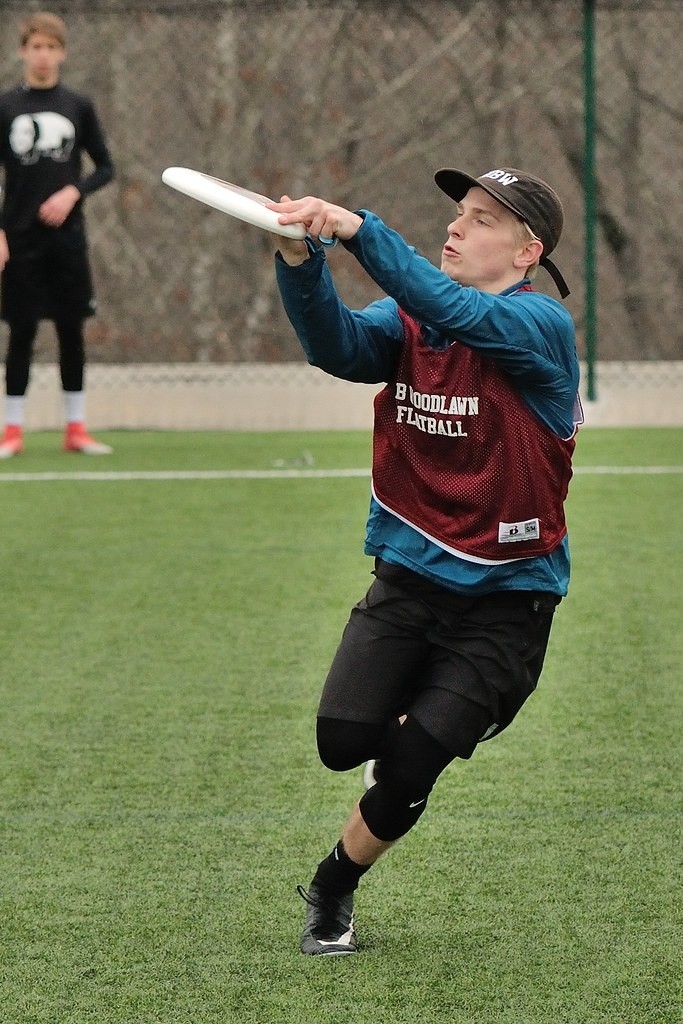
[434,167,571,300]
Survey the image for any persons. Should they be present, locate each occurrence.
[0,13,116,459]
[263,167,586,958]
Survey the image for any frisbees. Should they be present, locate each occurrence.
[160,166,307,243]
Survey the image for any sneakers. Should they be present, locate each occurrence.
[297,858,357,956]
[64,421,114,456]
[0,424,24,457]
[364,760,378,789]
